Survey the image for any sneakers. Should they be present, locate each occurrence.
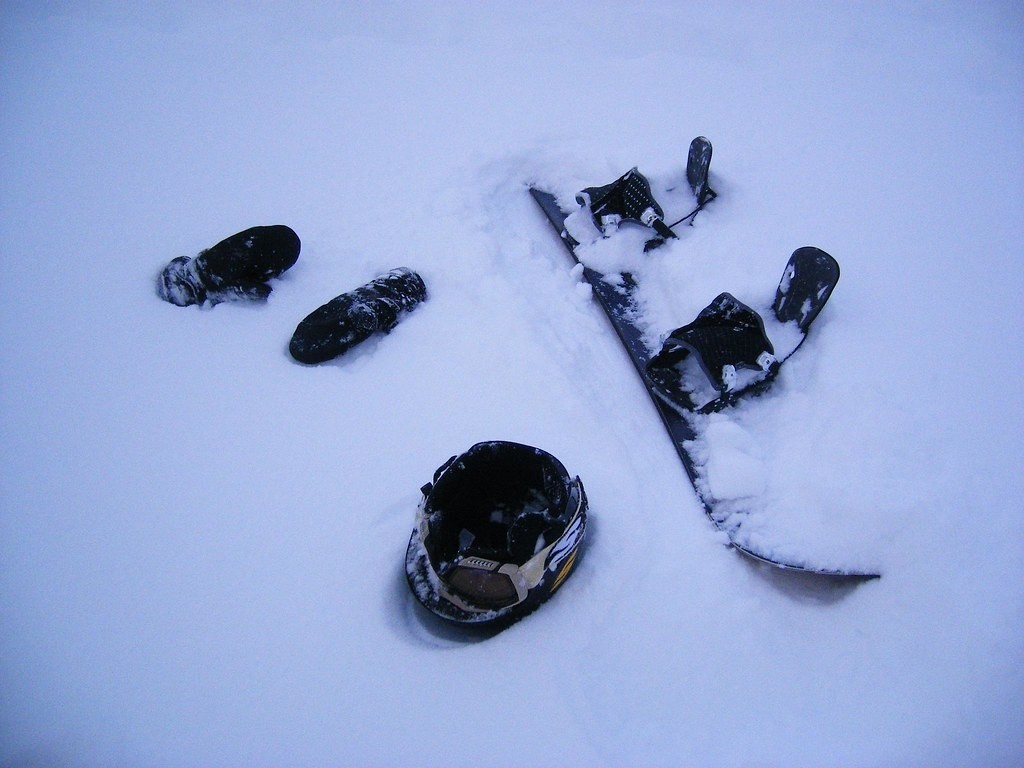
[291,266,428,364]
[158,226,301,306]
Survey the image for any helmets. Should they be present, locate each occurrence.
[406,438,589,627]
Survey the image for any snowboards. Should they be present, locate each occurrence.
[526,179,883,577]
